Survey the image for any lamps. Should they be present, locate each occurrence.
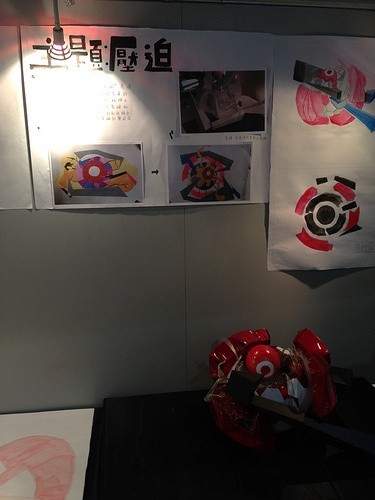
[45,0,74,61]
